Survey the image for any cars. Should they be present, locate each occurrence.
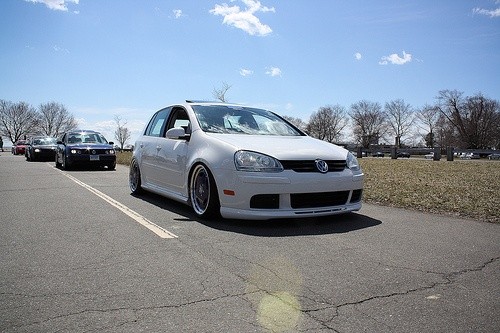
[11,139,27,155]
[128,98,364,224]
[55,129,117,170]
[25,134,57,161]
